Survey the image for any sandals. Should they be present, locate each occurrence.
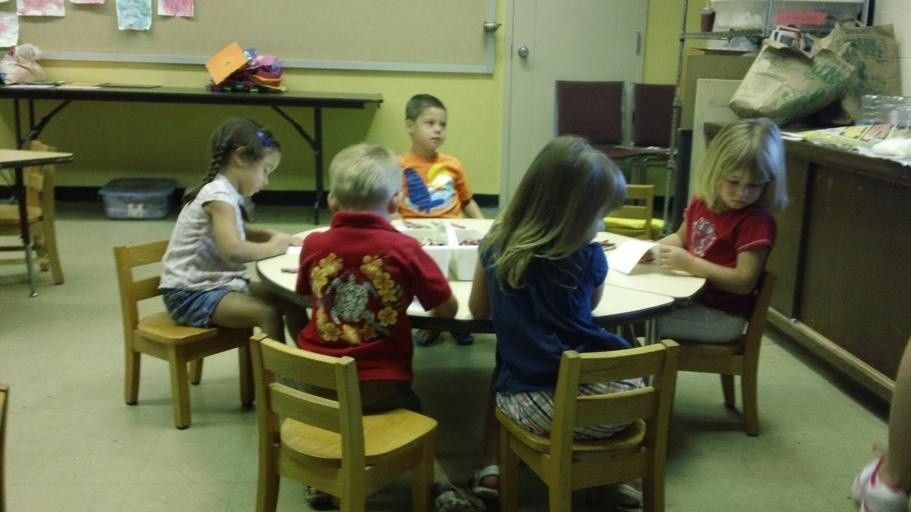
[617,483,643,512]
[464,465,501,499]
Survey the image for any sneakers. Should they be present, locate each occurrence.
[415,328,442,346]
[304,485,331,509]
[451,329,474,345]
[431,478,489,512]
[852,457,908,512]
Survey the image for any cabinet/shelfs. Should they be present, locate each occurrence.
[658,0,878,260]
[704,119,910,406]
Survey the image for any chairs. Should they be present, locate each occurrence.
[111,238,254,430]
[248,332,440,512]
[664,267,778,436]
[555,79,637,161]
[494,339,680,512]
[632,82,680,184]
[1,140,65,284]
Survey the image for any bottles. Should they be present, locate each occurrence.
[701,7,716,32]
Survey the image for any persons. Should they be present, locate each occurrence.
[849,331,911,510]
[472,136,649,510]
[293,144,456,511]
[390,95,483,345]
[641,117,788,346]
[154,116,309,345]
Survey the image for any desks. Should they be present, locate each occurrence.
[257,217,710,336]
[0,148,74,296]
[1,81,384,225]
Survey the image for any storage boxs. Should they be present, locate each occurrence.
[96,176,178,219]
